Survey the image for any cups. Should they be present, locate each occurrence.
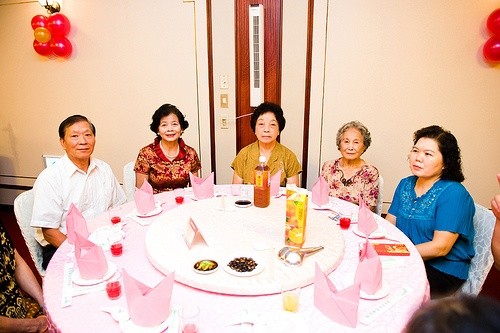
[177,304,200,333]
[106,233,123,258]
[281,283,301,313]
[174,188,185,206]
[339,210,353,231]
[102,271,122,300]
[230,185,241,196]
[108,205,122,225]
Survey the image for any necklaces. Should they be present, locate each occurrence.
[160,139,179,158]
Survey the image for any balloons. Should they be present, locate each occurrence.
[31,14,73,58]
[482,7,500,62]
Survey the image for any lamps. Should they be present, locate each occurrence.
[38,0,60,14]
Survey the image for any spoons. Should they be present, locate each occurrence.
[98,306,130,322]
[279,246,324,268]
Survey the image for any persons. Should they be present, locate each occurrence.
[0,219,49,333]
[230,101,303,188]
[319,120,380,214]
[132,104,202,195]
[30,115,128,271]
[384,125,476,299]
[476,173,500,302]
[402,291,500,333]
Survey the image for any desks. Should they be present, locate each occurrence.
[43,182,431,333]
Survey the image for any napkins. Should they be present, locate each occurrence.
[315,261,361,328]
[73,231,109,280]
[134,179,156,214]
[66,202,88,243]
[358,195,378,236]
[122,267,177,326]
[352,237,383,295]
[270,171,281,196]
[311,177,330,205]
[188,171,215,197]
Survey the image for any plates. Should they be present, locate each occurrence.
[119,309,169,333]
[70,261,117,286]
[279,187,308,198]
[233,199,253,208]
[349,281,390,300]
[352,224,388,238]
[84,230,125,248]
[135,206,163,217]
[190,192,218,201]
[309,198,338,209]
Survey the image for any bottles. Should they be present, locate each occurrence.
[254,156,271,209]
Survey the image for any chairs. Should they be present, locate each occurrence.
[14,187,46,277]
[123,160,136,198]
[429,203,494,300]
